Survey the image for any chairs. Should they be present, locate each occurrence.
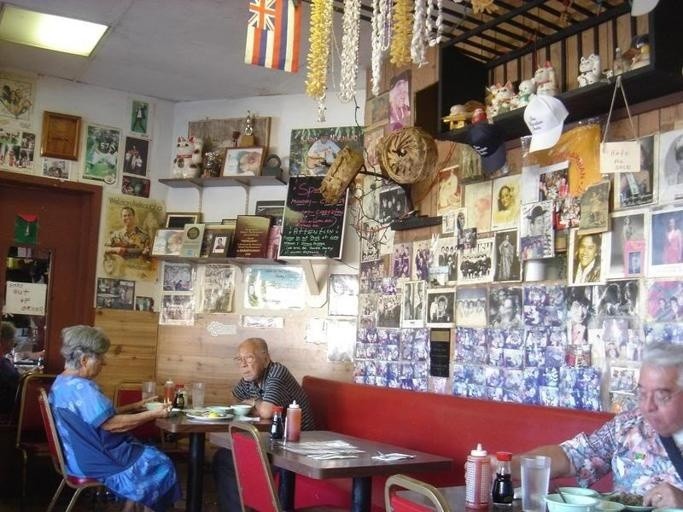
[0,342,598,510]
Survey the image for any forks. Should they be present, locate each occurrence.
[375,449,416,458]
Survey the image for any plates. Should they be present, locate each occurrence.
[206,405,230,412]
[167,408,180,413]
[598,488,681,512]
[186,413,233,420]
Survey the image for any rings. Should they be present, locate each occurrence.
[656,492,663,499]
[166,407,171,413]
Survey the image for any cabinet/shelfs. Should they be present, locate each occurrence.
[429,0,683,153]
[149,174,286,268]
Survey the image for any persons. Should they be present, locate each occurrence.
[306,130,341,175]
[387,80,409,122]
[6,88,25,114]
[105,207,152,264]
[213,337,319,511]
[134,103,147,133]
[364,185,497,330]
[497,148,682,326]
[215,237,224,249]
[47,325,181,511]
[0,321,39,423]
[461,344,682,511]
[126,145,142,168]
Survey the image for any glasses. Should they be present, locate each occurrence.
[632,385,682,400]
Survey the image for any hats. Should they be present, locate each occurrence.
[467,124,506,175]
[527,207,547,219]
[524,95,570,153]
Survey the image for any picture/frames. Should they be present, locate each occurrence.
[39,107,83,163]
[161,211,202,230]
[207,231,231,257]
[219,145,267,178]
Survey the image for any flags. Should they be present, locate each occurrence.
[244,1,302,74]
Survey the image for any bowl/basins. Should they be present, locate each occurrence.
[229,404,251,416]
[145,402,163,412]
[554,486,599,497]
[544,492,598,512]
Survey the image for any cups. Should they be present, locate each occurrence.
[190,381,204,409]
[518,453,551,511]
[140,380,156,402]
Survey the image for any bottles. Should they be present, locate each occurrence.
[489,450,516,512]
[464,443,491,510]
[269,406,283,439]
[283,399,302,442]
[163,378,188,409]
